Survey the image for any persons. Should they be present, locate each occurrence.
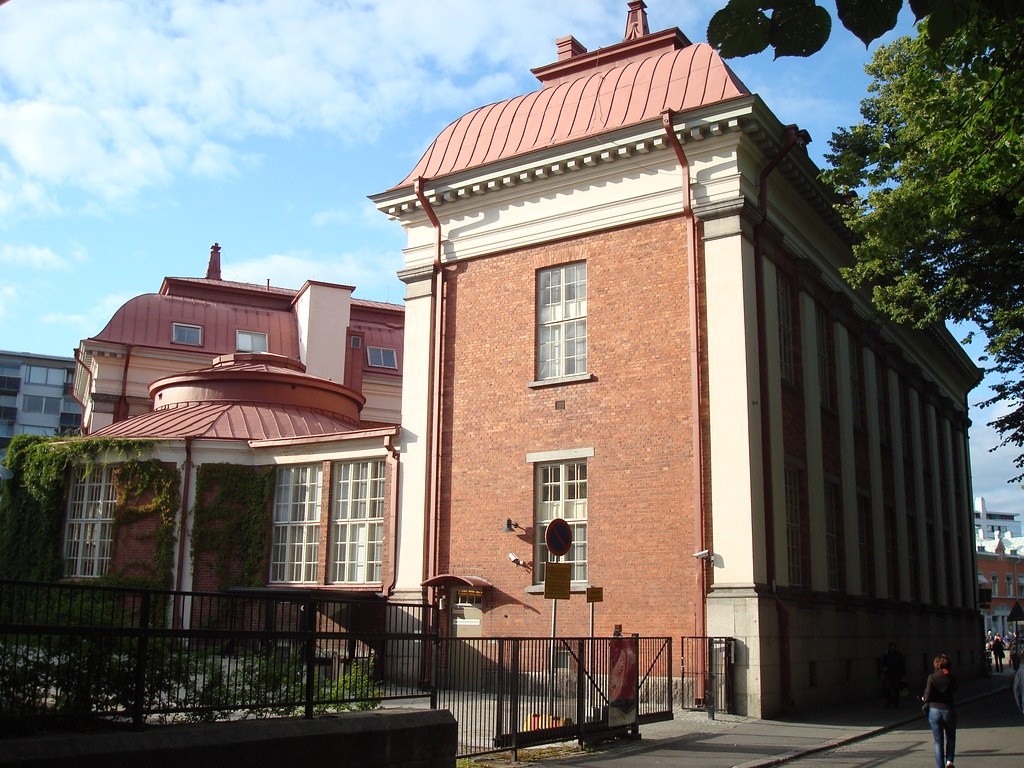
[923,654,958,768]
[1013,664,1024,718]
[991,639,1005,673]
[880,642,906,710]
[1010,640,1019,673]
[988,631,1010,649]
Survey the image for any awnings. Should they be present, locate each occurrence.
[420,574,493,589]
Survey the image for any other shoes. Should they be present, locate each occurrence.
[946,761,955,768]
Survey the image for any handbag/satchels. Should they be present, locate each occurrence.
[922,702,929,717]
[1001,652,1005,658]
